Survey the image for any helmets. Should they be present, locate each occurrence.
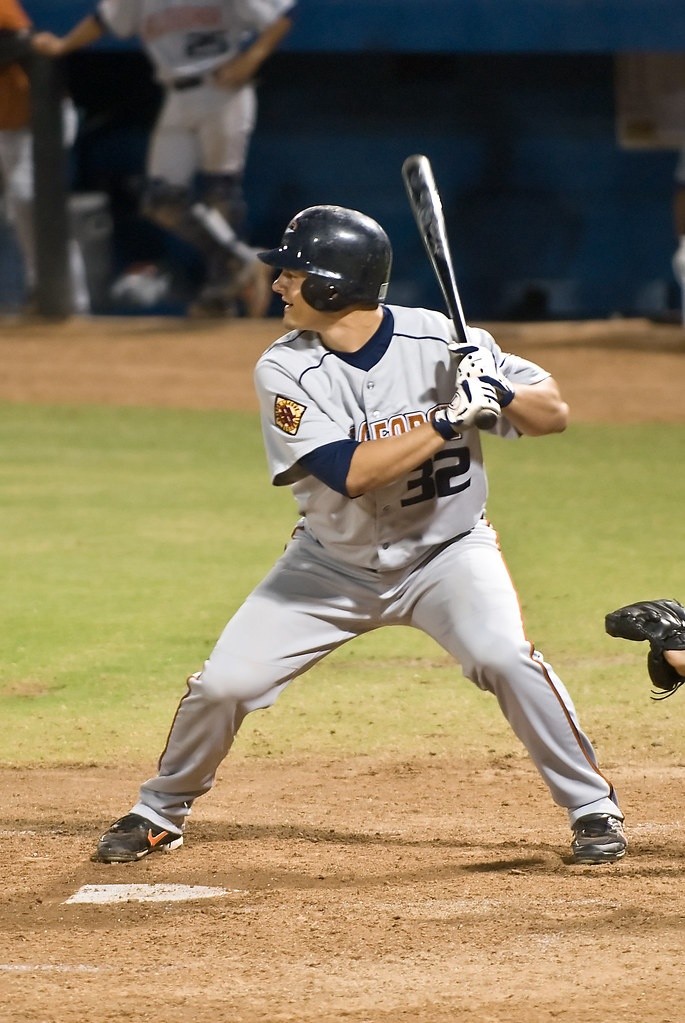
[257,205,393,312]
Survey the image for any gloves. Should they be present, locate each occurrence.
[431,376,501,441]
[447,343,515,408]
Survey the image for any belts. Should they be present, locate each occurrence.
[175,77,199,90]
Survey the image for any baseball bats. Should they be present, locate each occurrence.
[402,153,498,430]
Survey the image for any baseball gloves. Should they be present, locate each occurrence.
[605,599,685,690]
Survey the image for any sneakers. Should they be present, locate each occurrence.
[97,814,184,861]
[571,813,628,863]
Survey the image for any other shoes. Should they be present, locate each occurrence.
[241,250,272,315]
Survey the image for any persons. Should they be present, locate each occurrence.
[0,0,66,299]
[88,204,626,862]
[33,2,290,320]
